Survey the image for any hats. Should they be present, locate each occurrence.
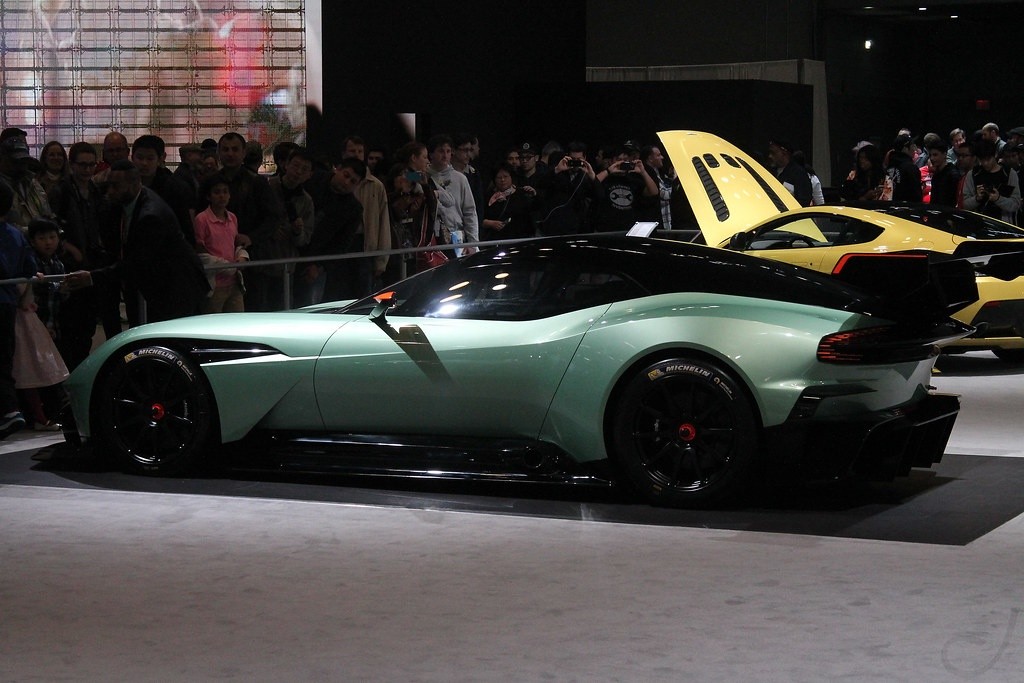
[517,141,538,154]
[624,140,640,151]
[852,140,874,151]
[893,133,920,149]
[179,145,206,157]
[1,137,30,160]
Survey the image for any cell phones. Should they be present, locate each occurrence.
[620,162,635,170]
[567,160,583,167]
[502,218,511,225]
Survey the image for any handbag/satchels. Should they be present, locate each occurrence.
[416,204,449,273]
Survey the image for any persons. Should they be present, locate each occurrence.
[0,117,1024,441]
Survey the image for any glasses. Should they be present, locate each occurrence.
[72,161,98,170]
[457,147,475,153]
[957,153,973,158]
[518,155,537,161]
[289,161,312,174]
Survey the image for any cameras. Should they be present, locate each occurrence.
[983,184,994,193]
[405,172,422,182]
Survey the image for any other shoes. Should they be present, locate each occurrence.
[34,419,62,431]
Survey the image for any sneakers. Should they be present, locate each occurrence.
[0,411,26,440]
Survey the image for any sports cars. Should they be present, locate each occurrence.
[656,130,1023,369]
[58,229,959,511]
